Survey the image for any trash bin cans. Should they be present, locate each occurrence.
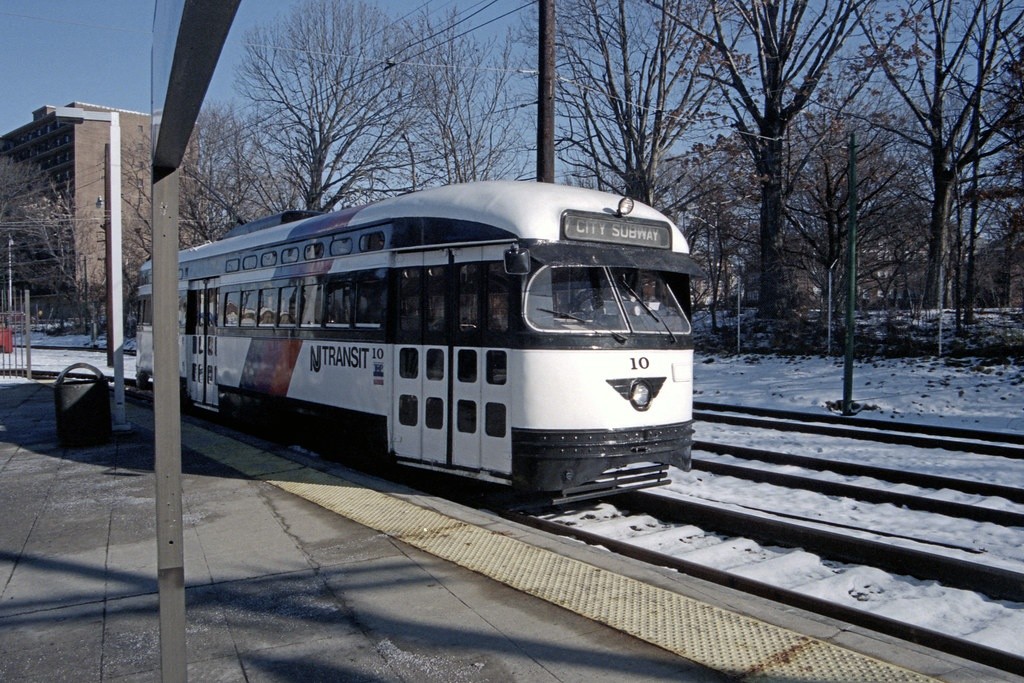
[53,362,113,449]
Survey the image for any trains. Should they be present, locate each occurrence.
[135,179,707,518]
